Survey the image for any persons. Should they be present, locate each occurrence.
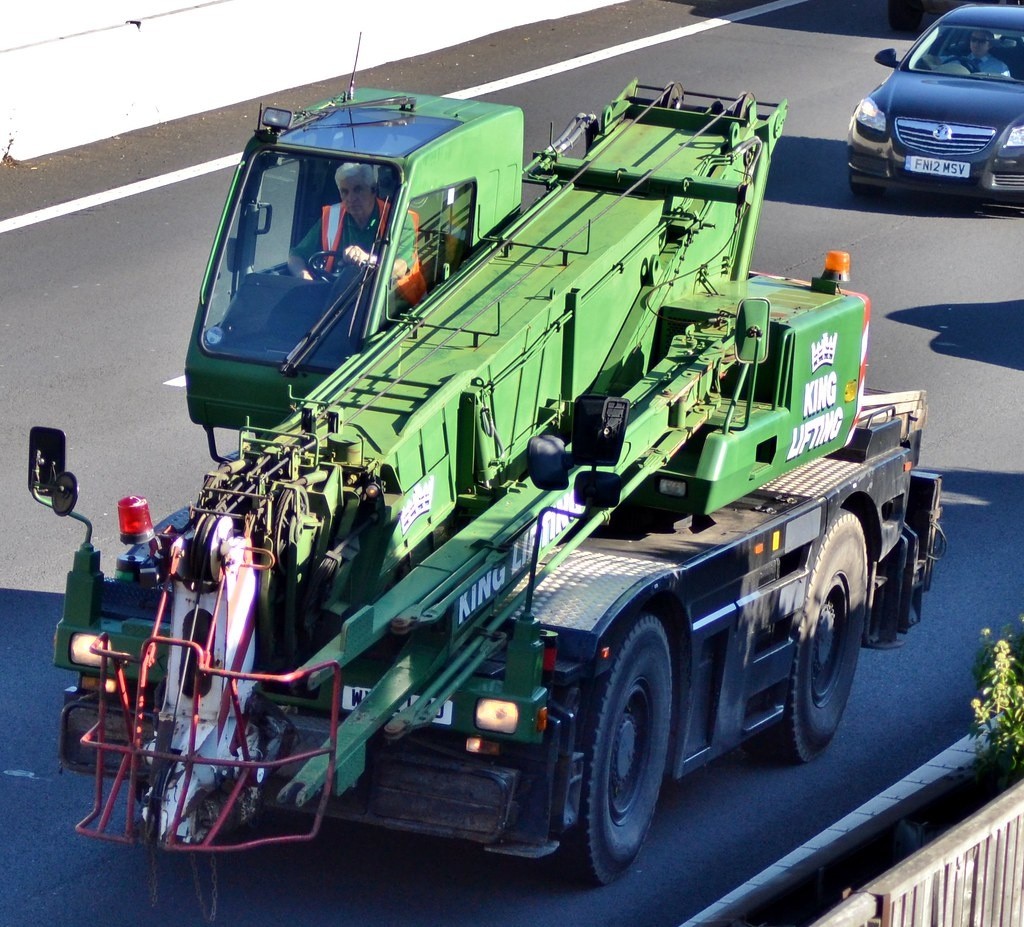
[921,30,1011,76]
[287,162,424,309]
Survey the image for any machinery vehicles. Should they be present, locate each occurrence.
[25,79,944,890]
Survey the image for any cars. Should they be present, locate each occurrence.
[847,3,1024,210]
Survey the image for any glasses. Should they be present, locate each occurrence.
[971,37,990,43]
